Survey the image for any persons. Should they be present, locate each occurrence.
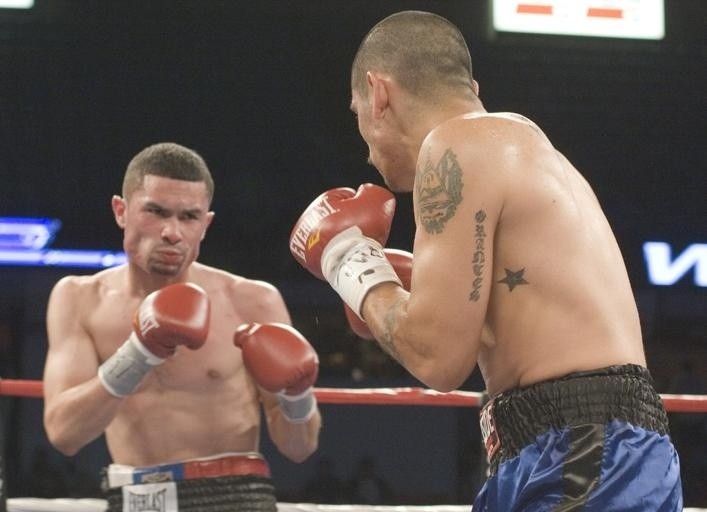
[40,142,324,512]
[286,7,684,512]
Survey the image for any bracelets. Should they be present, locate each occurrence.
[323,229,395,322]
[97,344,153,400]
[278,388,316,422]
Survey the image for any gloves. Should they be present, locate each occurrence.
[343,248,414,341]
[288,183,405,324]
[233,322,320,424]
[97,281,211,399]
